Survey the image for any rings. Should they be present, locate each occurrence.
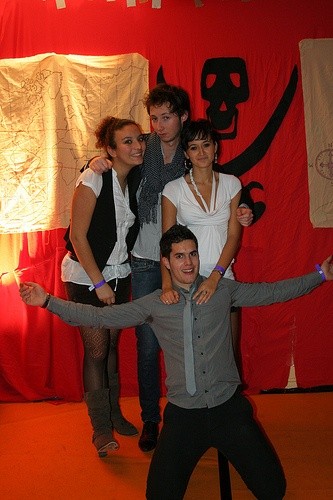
[165,300,168,303]
[201,291,207,294]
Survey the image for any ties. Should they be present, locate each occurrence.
[179,285,197,397]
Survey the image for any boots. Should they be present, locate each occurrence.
[85,390,119,458]
[109,375,138,436]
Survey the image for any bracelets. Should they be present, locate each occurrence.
[41,294,50,308]
[88,279,106,291]
[212,265,225,276]
[314,264,326,281]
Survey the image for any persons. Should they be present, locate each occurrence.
[79,83,253,452]
[160,119,242,376]
[19,225,333,500]
[61,115,146,457]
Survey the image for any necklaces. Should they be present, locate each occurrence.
[121,178,126,189]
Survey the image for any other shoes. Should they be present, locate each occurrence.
[138,421,159,451]
